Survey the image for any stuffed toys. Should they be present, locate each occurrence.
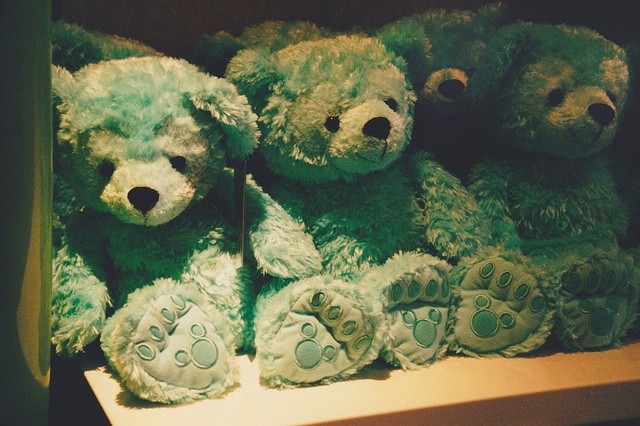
[52,17,173,245]
[402,1,529,165]
[186,19,377,65]
[225,18,557,393]
[493,23,636,350]
[50,56,386,403]
